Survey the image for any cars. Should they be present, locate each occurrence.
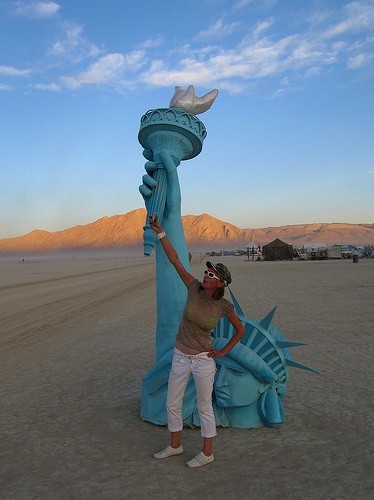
[218,252,222,256]
[233,252,240,256]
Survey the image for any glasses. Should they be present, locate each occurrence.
[203,270,221,281]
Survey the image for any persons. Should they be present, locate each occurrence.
[148,214,244,468]
[139,149,287,429]
[189,253,192,263]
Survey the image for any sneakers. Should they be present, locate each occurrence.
[153,444,183,459]
[186,451,214,467]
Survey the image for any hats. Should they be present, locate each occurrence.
[206,261,232,287]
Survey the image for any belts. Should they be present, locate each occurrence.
[174,351,207,359]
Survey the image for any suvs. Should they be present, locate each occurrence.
[342,247,365,260]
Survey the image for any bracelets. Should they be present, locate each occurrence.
[156,231,166,240]
